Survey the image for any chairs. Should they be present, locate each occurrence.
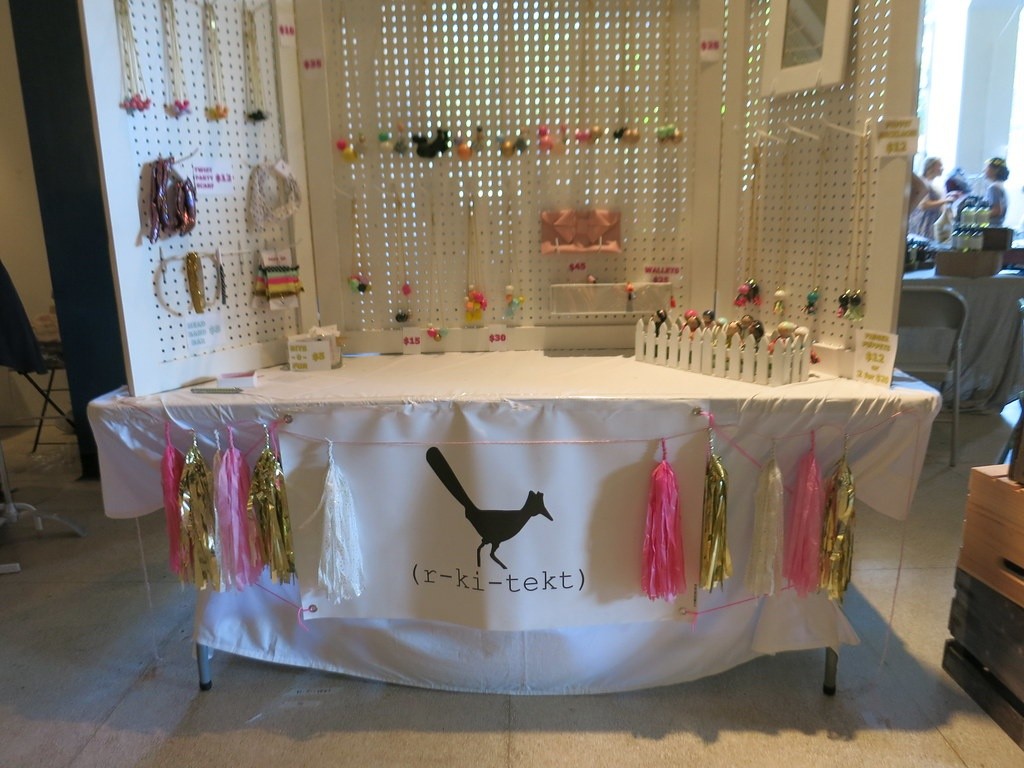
[895,284,965,468]
[0,260,77,451]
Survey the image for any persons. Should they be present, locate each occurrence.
[981,158,1009,227]
[906,156,954,241]
[653,308,820,367]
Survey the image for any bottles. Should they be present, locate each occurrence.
[961,200,993,228]
[951,224,984,251]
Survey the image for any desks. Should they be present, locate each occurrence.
[898,268,1024,414]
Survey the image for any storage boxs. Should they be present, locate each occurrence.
[286,335,341,371]
[218,370,258,389]
[935,228,1024,275]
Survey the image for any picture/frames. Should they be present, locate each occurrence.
[762,0,855,96]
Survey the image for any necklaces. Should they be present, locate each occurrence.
[771,155,790,316]
[492,0,530,156]
[241,8,269,122]
[347,202,368,291]
[411,0,448,158]
[732,149,762,308]
[454,0,485,161]
[535,1,568,154]
[503,198,526,318]
[464,200,487,312]
[612,0,641,143]
[836,134,865,321]
[377,0,408,156]
[800,148,823,318]
[573,0,603,142]
[336,6,368,162]
[657,0,681,143]
[115,0,150,111]
[427,199,448,341]
[394,202,410,323]
[158,0,190,117]
[201,0,228,120]
